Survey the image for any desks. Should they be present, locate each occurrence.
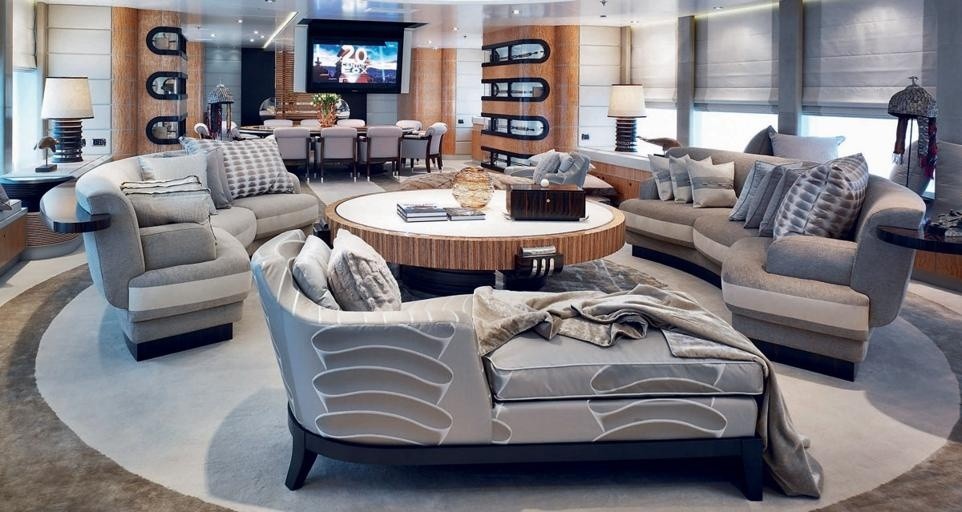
[1,154,112,264]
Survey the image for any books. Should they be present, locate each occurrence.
[397,202,448,218]
[443,206,486,221]
[397,210,448,223]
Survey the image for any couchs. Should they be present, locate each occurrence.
[614,144,926,383]
[249,225,773,493]
[71,151,322,362]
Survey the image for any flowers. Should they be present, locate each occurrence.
[309,92,342,117]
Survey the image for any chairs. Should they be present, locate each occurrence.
[194,118,449,185]
[505,150,592,188]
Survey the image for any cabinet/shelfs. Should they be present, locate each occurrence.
[589,160,614,188]
[614,165,651,207]
[0,212,27,269]
[472,123,483,161]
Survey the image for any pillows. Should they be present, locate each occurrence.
[526,148,556,164]
[743,124,777,155]
[328,226,404,313]
[533,152,561,184]
[557,152,573,172]
[767,129,846,162]
[120,135,300,243]
[890,139,932,197]
[288,231,343,311]
[644,152,873,244]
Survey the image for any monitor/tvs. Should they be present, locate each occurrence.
[305,34,403,95]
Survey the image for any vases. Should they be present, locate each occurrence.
[315,104,338,129]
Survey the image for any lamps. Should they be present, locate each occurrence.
[38,75,96,165]
[606,82,648,153]
[887,74,938,186]
